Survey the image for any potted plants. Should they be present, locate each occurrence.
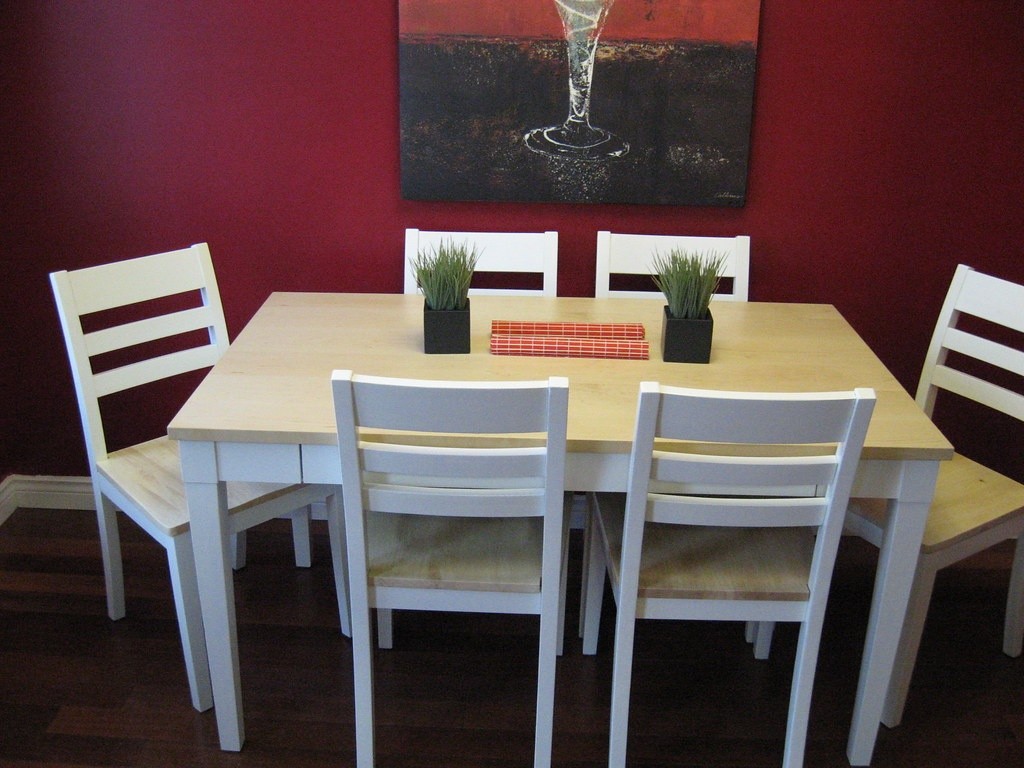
[646,244,730,364]
[409,233,485,353]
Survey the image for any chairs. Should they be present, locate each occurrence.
[48,240,350,712]
[326,369,572,768]
[753,263,1024,725]
[579,231,750,636]
[583,380,878,768]
[404,228,559,298]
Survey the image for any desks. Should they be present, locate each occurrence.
[164,290,957,765]
